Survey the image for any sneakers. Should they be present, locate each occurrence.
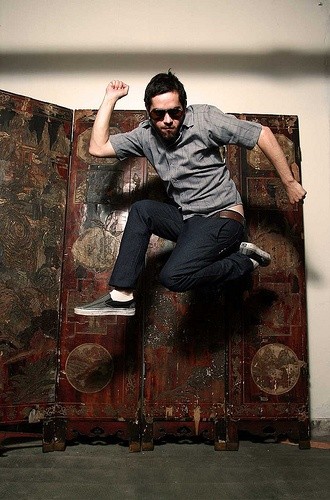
[240,242,272,268]
[73,294,136,317]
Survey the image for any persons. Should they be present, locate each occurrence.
[73,67,305,316]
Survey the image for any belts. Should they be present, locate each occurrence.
[210,210,246,225]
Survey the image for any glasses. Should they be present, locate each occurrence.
[148,109,185,121]
